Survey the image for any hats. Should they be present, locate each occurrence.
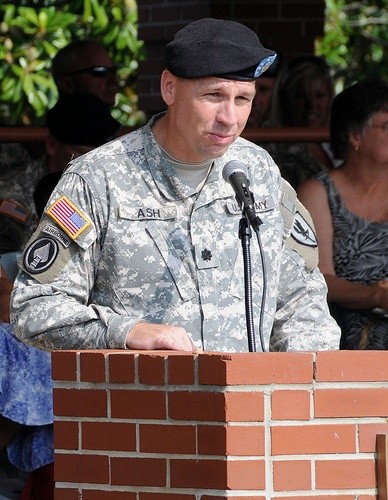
[164,18,277,81]
[46,93,121,147]
[33,170,64,218]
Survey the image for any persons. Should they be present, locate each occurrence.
[1,36,336,500]
[297,77,388,350]
[9,17,341,351]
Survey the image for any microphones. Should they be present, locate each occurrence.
[222,159,259,232]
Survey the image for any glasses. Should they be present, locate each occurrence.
[79,65,117,77]
[371,121,388,133]
